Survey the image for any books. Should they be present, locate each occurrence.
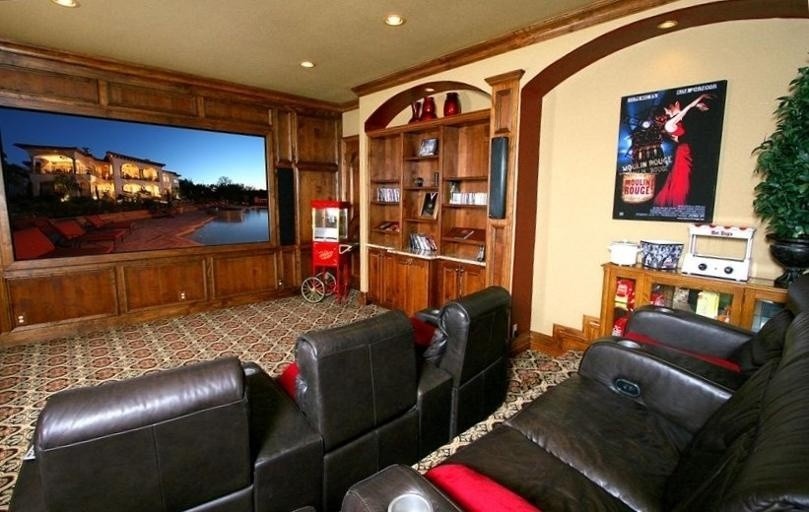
[448,192,488,206]
[409,231,437,252]
[375,186,401,203]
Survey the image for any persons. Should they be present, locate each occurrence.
[646,91,717,208]
[660,92,715,217]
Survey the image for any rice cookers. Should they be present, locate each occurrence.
[608,238,644,267]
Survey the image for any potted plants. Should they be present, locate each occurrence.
[747,62,809,287]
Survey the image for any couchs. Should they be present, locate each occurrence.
[8,284,809,512]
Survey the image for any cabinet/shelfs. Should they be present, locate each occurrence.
[364,106,493,319]
[599,260,791,342]
